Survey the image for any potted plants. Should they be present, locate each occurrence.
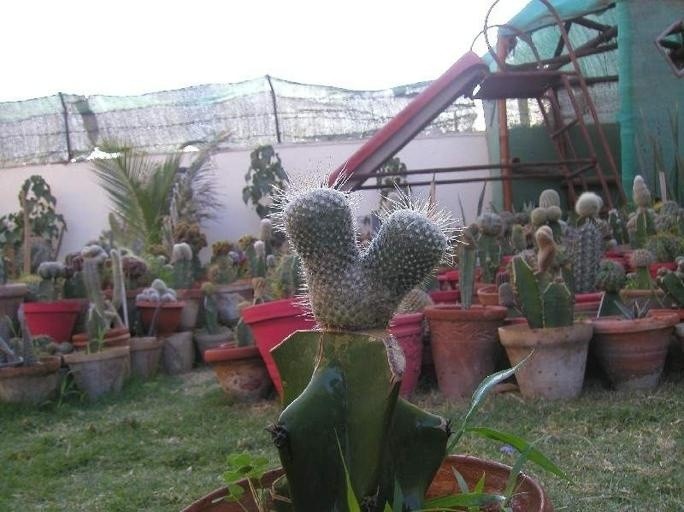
[183,185,549,510]
[0,122,683,404]
